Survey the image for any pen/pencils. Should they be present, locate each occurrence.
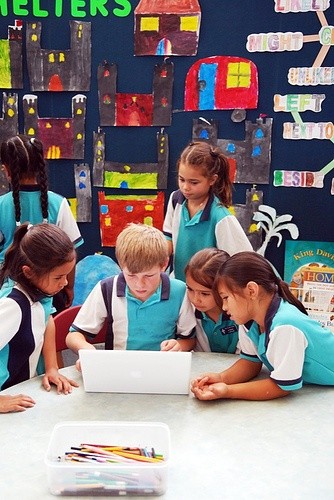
[53,443,165,496]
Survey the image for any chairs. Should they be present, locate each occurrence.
[54,304,109,369]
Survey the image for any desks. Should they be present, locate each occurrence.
[0,352,334,500]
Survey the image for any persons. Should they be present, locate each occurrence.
[0,221,81,413]
[182,247,243,354]
[66,220,199,372]
[190,251,334,407]
[158,138,256,294]
[0,133,86,281]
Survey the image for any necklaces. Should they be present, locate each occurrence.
[185,201,203,222]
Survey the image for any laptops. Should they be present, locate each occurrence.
[78,349,192,395]
